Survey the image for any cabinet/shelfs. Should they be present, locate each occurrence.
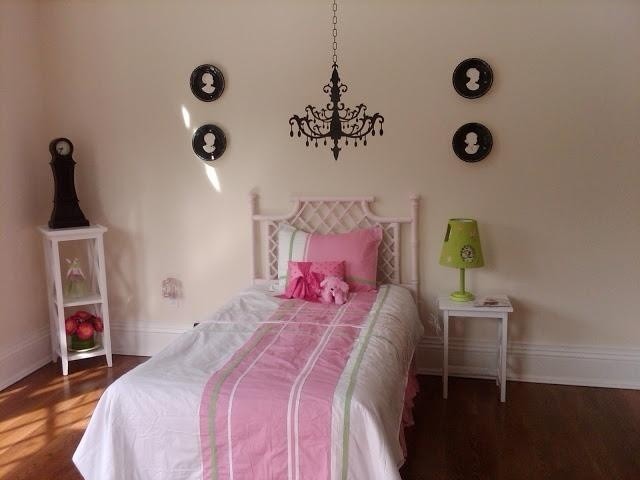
[34,224,116,377]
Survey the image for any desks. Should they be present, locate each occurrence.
[436,294,514,403]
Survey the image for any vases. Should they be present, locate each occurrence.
[71,333,94,350]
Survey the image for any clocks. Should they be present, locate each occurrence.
[46,137,89,229]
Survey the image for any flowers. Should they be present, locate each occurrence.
[66,309,103,340]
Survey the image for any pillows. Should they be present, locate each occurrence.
[286,260,347,293]
[275,221,383,294]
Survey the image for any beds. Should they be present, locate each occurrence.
[70,187,428,480]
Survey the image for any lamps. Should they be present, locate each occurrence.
[438,217,484,302]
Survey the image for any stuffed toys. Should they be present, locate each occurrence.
[321,276,349,304]
